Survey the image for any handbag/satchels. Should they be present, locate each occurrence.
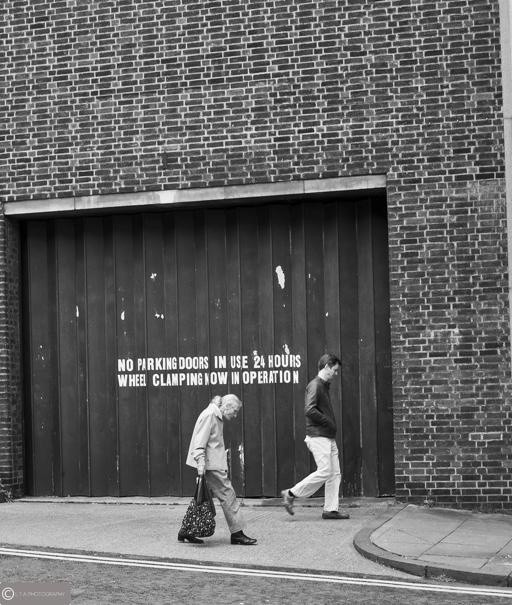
[180,474,217,536]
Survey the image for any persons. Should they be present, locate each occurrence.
[178,393,258,544]
[281,353,350,519]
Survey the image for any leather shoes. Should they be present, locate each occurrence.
[178,532,204,544]
[230,530,258,545]
[322,510,350,519]
[280,489,295,516]
